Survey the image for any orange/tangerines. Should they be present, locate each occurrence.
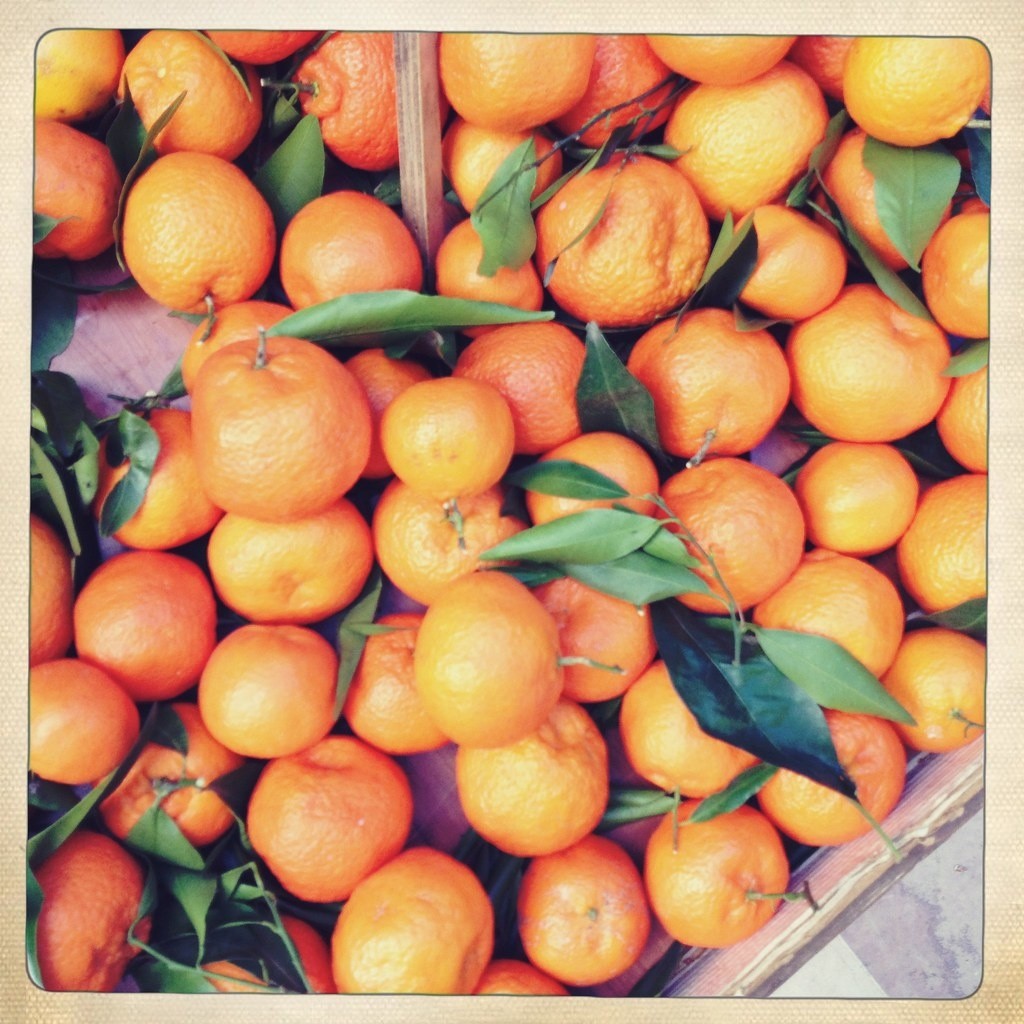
[31,30,990,998]
